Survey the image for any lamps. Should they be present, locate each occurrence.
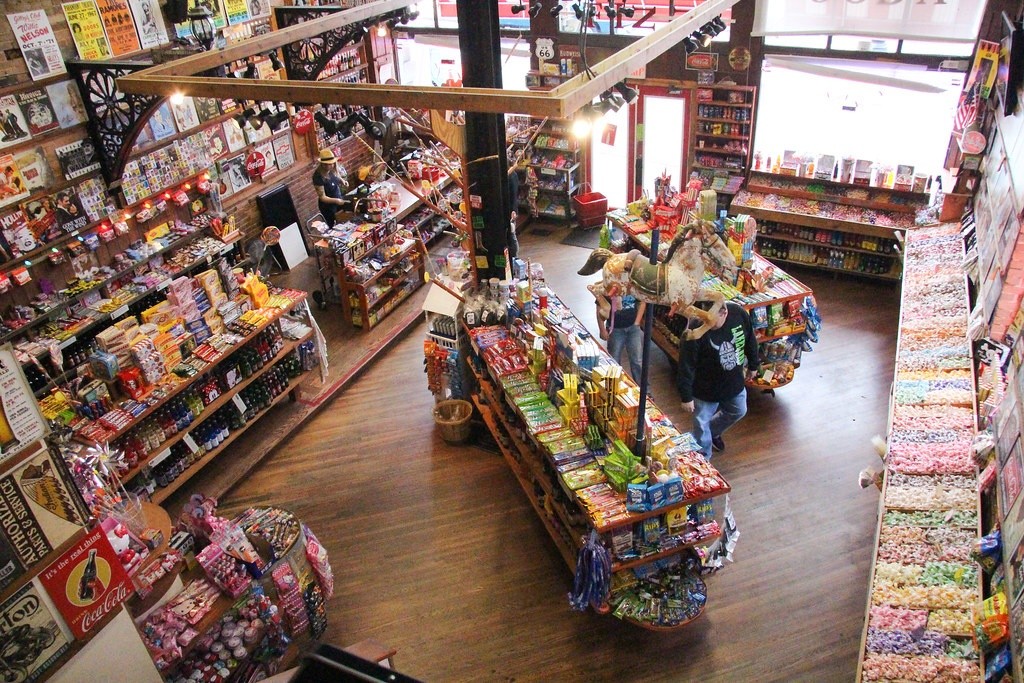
[386,9,419,29]
[683,14,726,55]
[581,81,639,123]
[214,49,284,80]
[187,0,215,52]
[511,0,634,19]
[352,27,368,44]
[233,99,387,140]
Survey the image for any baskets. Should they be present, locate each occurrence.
[434,399,472,443]
[573,193,608,228]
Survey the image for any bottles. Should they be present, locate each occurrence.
[311,47,367,83]
[753,218,894,275]
[754,151,933,193]
[8,308,22,320]
[26,323,318,494]
[560,58,578,76]
[353,191,401,223]
[86,286,170,322]
[229,242,241,266]
[80,549,98,601]
[303,103,366,150]
[698,105,750,136]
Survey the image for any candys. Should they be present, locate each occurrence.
[731,174,925,229]
[862,222,984,683]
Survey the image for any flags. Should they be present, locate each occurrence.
[955,85,979,130]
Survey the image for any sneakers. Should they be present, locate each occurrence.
[712,435,724,451]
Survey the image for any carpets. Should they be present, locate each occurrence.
[560,226,604,249]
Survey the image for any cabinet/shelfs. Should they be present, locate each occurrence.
[396,129,439,151]
[314,166,462,332]
[690,84,756,209]
[460,293,732,614]
[43,291,320,507]
[605,209,813,396]
[507,74,587,237]
[727,169,931,283]
[1,216,246,401]
[128,505,330,683]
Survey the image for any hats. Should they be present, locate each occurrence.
[317,148,339,163]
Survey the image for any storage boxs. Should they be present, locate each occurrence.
[507,257,684,512]
[139,528,252,600]
[78,267,268,408]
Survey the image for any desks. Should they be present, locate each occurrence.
[126,501,172,577]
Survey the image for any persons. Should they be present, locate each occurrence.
[73,23,83,39]
[596,296,655,400]
[313,149,351,228]
[98,39,109,55]
[140,4,157,36]
[677,299,758,462]
[27,50,48,77]
[56,194,81,232]
[507,159,520,279]
[26,197,49,221]
[0,138,97,202]
[265,151,275,168]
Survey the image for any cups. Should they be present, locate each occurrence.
[699,141,705,148]
[119,492,150,533]
[446,252,548,309]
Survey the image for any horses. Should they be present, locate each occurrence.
[577,220,736,342]
